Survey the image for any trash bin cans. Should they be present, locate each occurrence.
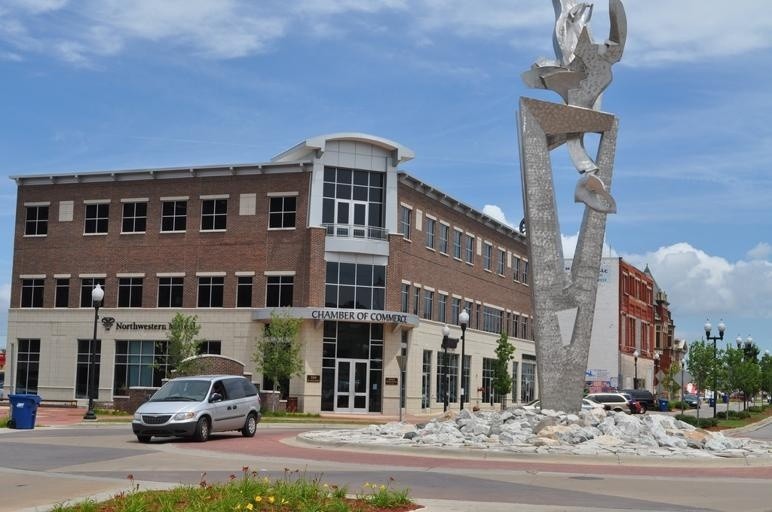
[658,398,669,411]
[709,399,715,407]
[287,397,297,413]
[7,394,41,429]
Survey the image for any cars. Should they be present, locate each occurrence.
[684,394,701,409]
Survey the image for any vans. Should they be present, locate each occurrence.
[522,389,655,415]
[132,374,261,442]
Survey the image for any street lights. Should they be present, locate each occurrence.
[654,352,659,386]
[633,350,640,389]
[681,358,686,414]
[84,283,104,419]
[704,317,725,419]
[458,309,470,410]
[442,324,451,412]
[736,334,752,411]
